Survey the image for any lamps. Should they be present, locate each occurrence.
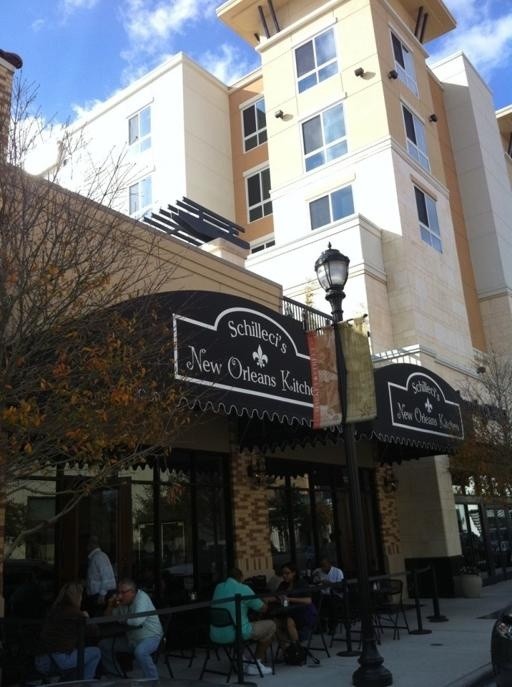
[274,109,284,120]
[388,70,399,80]
[353,67,366,79]
[429,113,438,122]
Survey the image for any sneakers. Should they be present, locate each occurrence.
[246,657,273,675]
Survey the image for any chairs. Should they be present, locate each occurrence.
[371,579,409,641]
[196,607,265,682]
[13,610,86,686]
[123,613,174,680]
[299,590,332,665]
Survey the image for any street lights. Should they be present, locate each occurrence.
[313,240,393,686]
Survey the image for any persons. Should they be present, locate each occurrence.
[207,567,278,677]
[199,538,219,590]
[311,557,346,637]
[26,578,102,681]
[96,576,165,685]
[270,561,321,667]
[80,532,119,616]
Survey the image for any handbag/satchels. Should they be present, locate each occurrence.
[283,644,307,666]
